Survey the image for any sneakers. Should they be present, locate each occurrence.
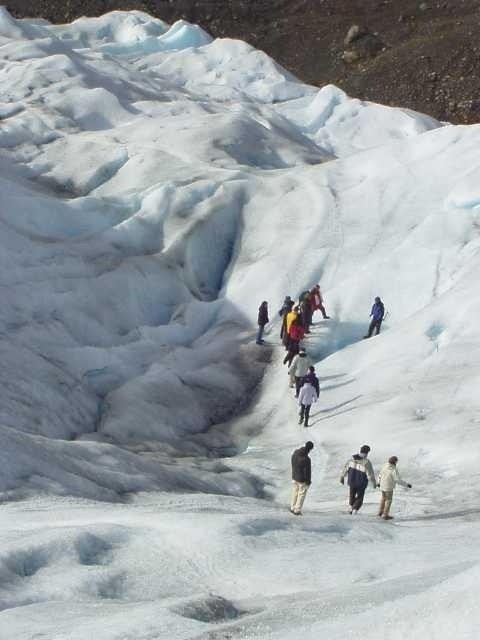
[291,510,303,516]
[294,394,299,399]
[347,506,361,516]
[309,323,314,326]
[304,328,311,333]
[288,384,294,388]
[298,412,309,427]
[363,336,372,339]
[323,316,330,319]
[377,513,393,520]
[256,339,264,344]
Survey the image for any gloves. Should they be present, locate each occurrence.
[408,484,412,488]
[283,361,286,364]
[377,484,380,487]
[369,314,372,317]
[287,372,290,375]
[340,478,344,485]
[373,486,376,489]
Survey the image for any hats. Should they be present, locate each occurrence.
[309,366,314,373]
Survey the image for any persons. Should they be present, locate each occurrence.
[256,300,270,345]
[339,445,378,515]
[363,297,386,338]
[278,283,334,426]
[375,456,414,520]
[290,441,315,515]
